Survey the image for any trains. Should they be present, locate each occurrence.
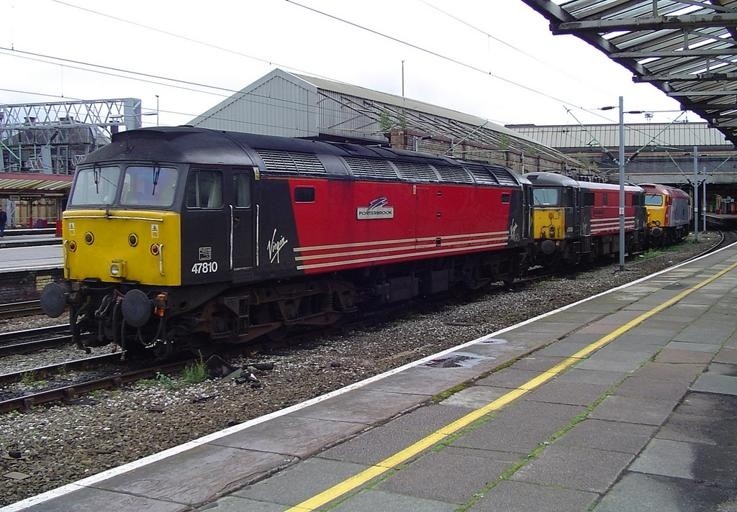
[37,111,695,360]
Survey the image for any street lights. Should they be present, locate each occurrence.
[600,92,645,275]
